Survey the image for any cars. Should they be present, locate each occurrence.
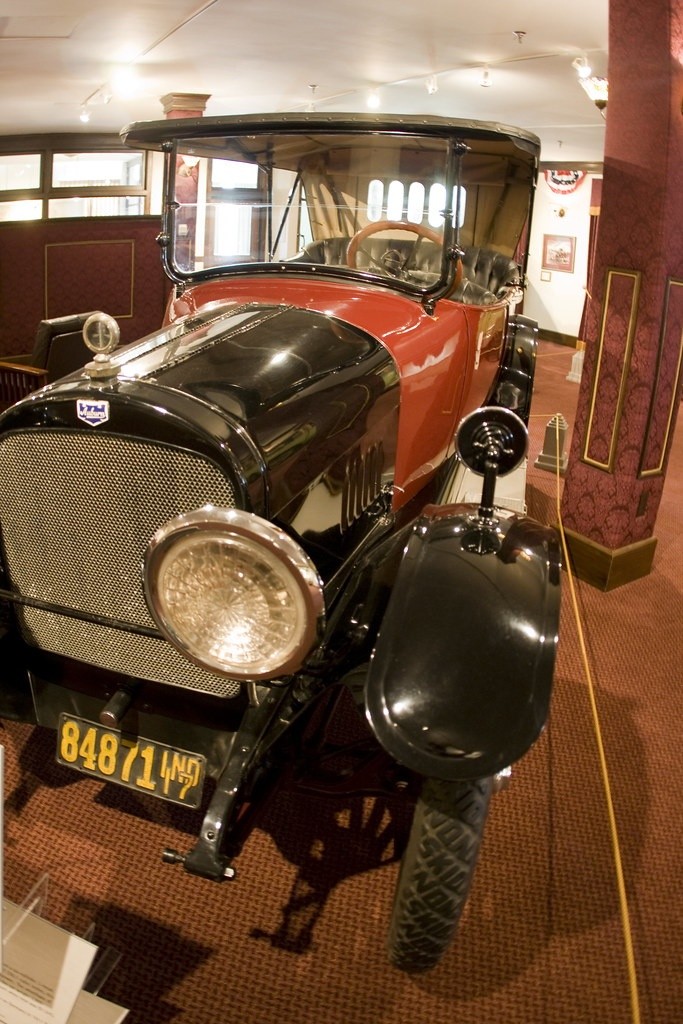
[2,109,563,977]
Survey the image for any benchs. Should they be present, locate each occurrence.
[284,237,517,300]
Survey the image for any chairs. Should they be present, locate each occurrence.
[0,310,102,412]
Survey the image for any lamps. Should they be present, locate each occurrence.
[573,58,608,119]
